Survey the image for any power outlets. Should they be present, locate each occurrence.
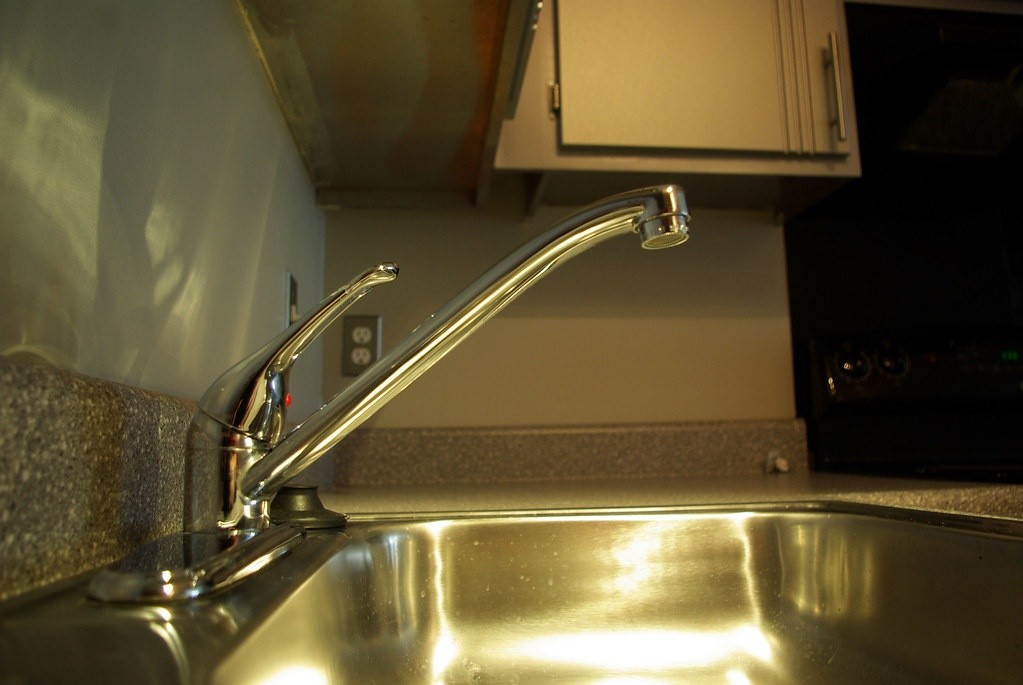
[286,273,300,326]
[341,316,384,377]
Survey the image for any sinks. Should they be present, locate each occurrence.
[182,506,1023,685]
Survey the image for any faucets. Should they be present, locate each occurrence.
[183,178,693,541]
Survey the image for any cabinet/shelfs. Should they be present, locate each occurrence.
[491,0,864,217]
